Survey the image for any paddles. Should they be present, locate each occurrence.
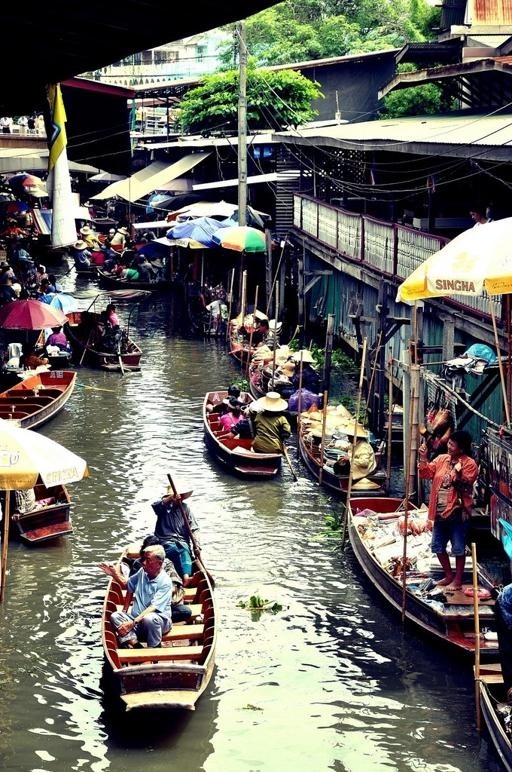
[472,543,482,727]
[118,341,124,375]
[167,473,215,590]
[284,445,298,482]
[65,264,75,274]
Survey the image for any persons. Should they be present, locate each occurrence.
[468,201,496,227]
[492,581,512,709]
[95,542,174,667]
[27,115,37,135]
[17,116,28,137]
[0,117,11,133]
[130,534,206,625]
[326,421,377,483]
[417,431,483,592]
[11,487,36,522]
[36,114,46,135]
[151,484,206,589]
[0,161,199,366]
[186,275,324,458]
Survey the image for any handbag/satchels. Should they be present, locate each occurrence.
[427,401,452,452]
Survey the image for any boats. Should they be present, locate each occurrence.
[71,219,162,293]
[95,535,221,716]
[1,474,72,546]
[0,368,78,432]
[59,299,148,373]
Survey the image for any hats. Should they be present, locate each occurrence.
[255,344,317,385]
[302,403,350,437]
[223,386,256,414]
[73,226,92,250]
[151,259,163,268]
[162,486,193,499]
[351,478,382,490]
[257,392,288,411]
[11,283,22,298]
[118,227,129,235]
[339,423,369,438]
[269,319,282,334]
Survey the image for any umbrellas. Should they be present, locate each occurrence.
[391,212,512,437]
[165,199,277,313]
[0,416,90,608]
